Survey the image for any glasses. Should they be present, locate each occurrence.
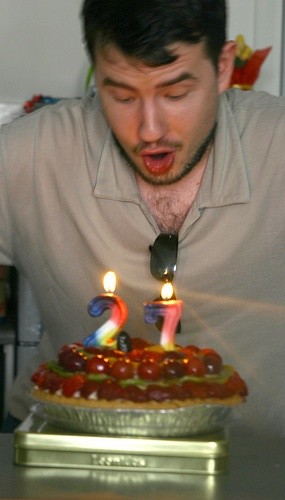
[150,233,182,335]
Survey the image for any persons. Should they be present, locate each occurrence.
[0,0,285,435]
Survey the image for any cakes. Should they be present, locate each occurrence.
[26,330,248,437]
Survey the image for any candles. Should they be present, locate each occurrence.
[145,283,183,350]
[87,271,132,354]
